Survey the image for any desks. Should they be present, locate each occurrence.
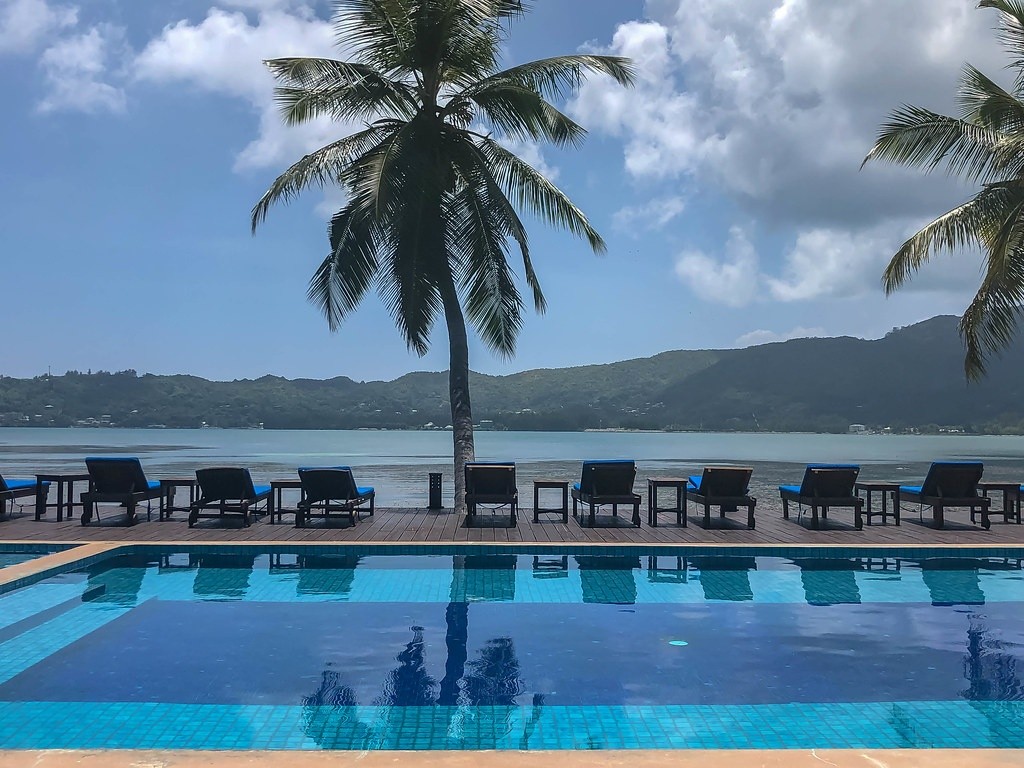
[159,476,200,521]
[854,481,901,526]
[534,480,569,523]
[270,479,310,523]
[971,482,1021,524]
[647,477,688,528]
[35,473,93,522]
[464,461,520,525]
[571,459,641,527]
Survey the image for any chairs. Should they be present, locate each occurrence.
[1004,484,1024,518]
[686,467,756,530]
[81,456,176,526]
[779,464,864,531]
[188,466,271,527]
[0,474,51,514]
[891,461,991,530]
[298,465,375,526]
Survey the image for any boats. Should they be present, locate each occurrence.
[583,426,668,433]
